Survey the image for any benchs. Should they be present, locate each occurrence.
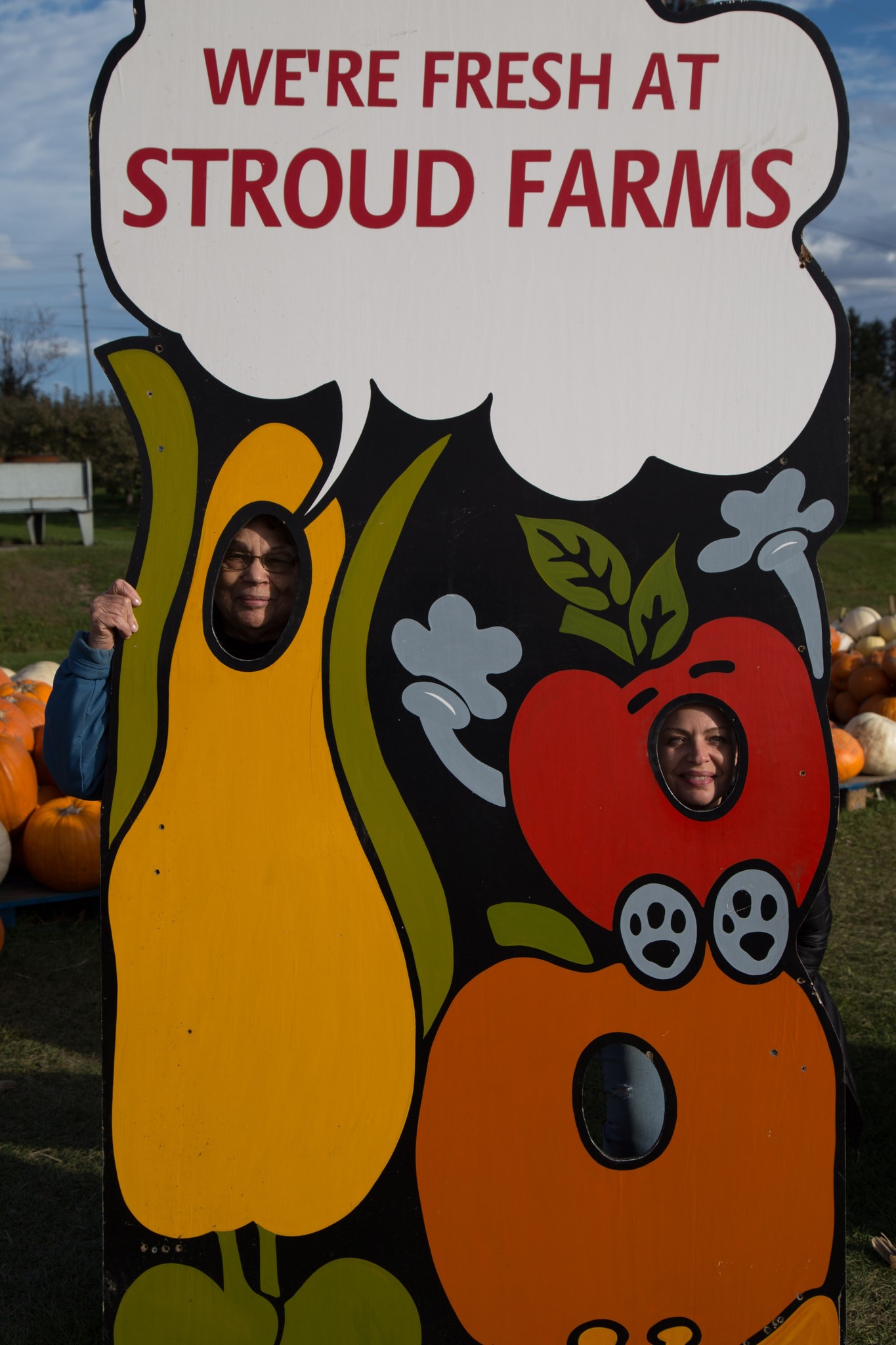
[0,459,93,546]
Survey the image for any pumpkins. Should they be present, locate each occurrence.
[827,592,895,781]
[0,662,102,889]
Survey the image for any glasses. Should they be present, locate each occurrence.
[221,549,302,575]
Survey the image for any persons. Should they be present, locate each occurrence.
[40,515,301,802]
[574,705,834,1159]
